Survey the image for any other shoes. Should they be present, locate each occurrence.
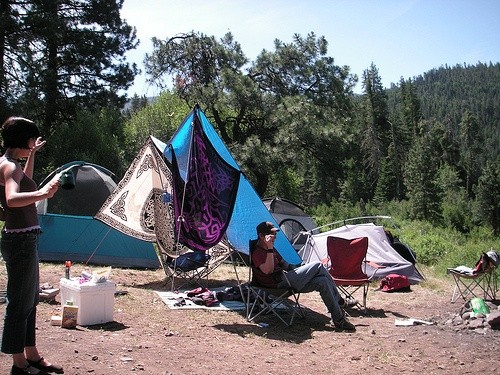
[339,300,358,309]
[335,320,355,330]
[10,363,46,375]
[26,358,63,372]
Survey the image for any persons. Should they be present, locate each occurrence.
[252,222,359,332]
[0,117,63,375]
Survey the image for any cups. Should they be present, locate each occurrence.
[81,271,92,279]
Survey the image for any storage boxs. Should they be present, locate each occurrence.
[62,306,78,329]
[60,278,116,327]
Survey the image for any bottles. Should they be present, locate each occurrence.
[65,261,71,279]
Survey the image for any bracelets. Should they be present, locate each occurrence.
[267,248,274,253]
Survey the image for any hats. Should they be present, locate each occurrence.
[257,222,280,235]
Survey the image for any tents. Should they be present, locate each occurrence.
[297,223,422,285]
[35,161,163,270]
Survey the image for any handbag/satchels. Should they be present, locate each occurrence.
[380,274,411,292]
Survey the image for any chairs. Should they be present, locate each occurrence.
[161,252,211,292]
[246,239,306,327]
[320,236,386,315]
[447,247,500,303]
